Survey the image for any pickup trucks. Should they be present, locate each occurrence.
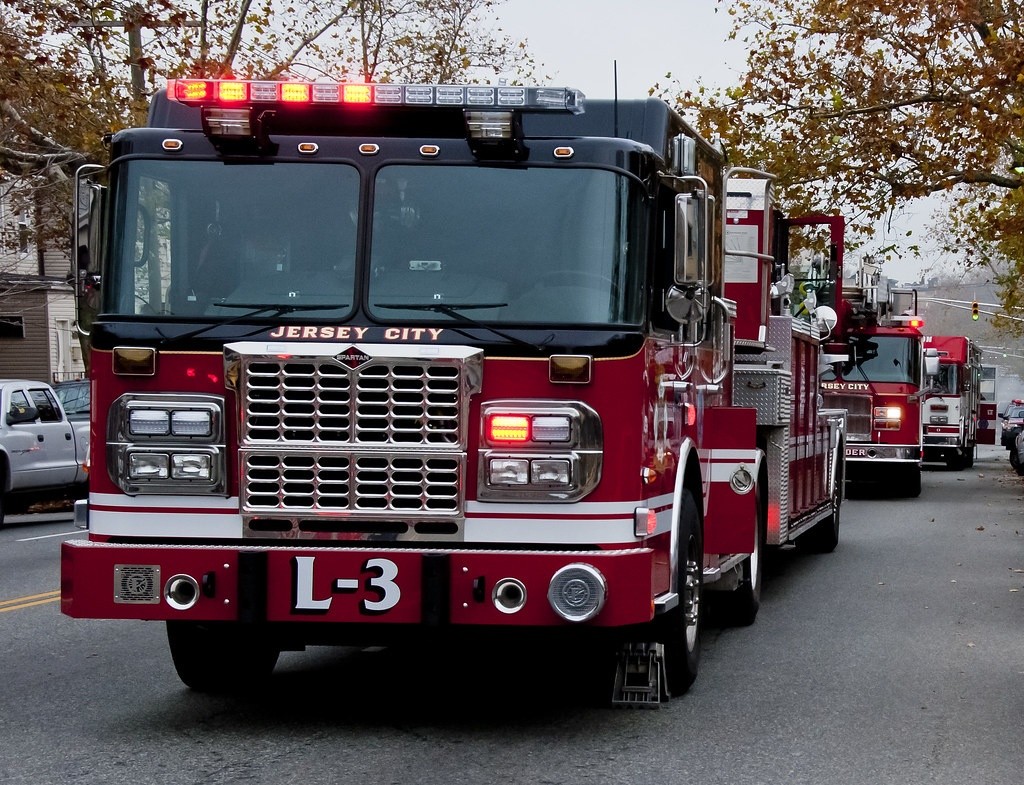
[1,379,91,527]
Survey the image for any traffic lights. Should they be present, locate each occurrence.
[971,302,979,322]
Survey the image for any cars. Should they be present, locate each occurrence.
[998,399,1023,478]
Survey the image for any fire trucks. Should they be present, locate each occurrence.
[61,78,849,710]
[816,254,930,499]
[922,335,997,471]
